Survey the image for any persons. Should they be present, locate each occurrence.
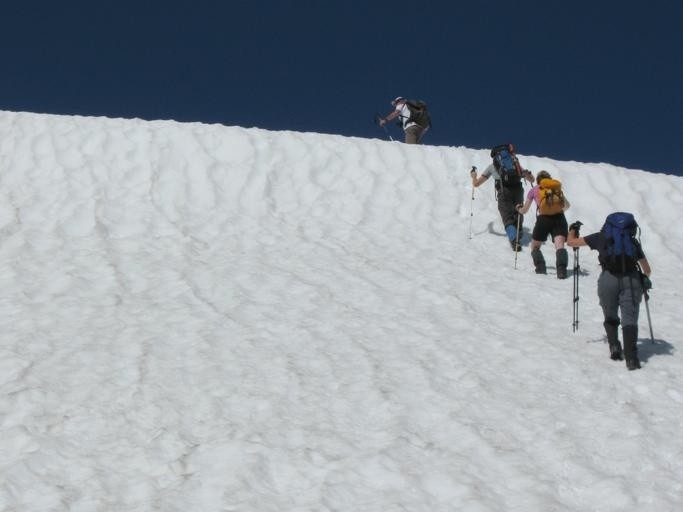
[379,96,427,144]
[565,215,651,370]
[514,170,572,280]
[470,142,535,252]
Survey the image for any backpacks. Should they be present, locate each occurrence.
[490,143,523,186]
[538,178,565,216]
[602,212,639,275]
[398,101,428,128]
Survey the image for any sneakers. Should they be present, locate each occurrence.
[512,238,522,251]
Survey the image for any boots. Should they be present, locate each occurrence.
[604,318,623,361]
[622,323,640,371]
[531,249,546,274]
[556,249,568,279]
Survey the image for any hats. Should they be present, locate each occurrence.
[391,97,404,107]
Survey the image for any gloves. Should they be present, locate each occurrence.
[569,223,580,231]
